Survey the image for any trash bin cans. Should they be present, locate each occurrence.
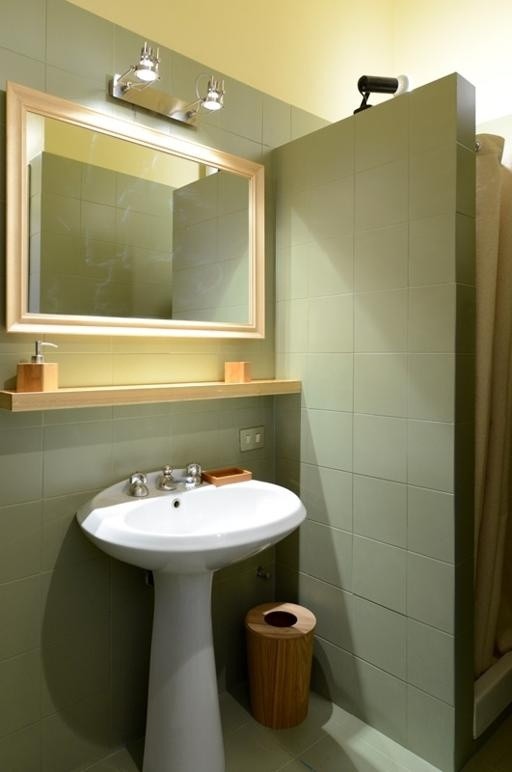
[244,600,319,729]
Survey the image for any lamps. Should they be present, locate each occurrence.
[110,41,226,130]
[352,75,398,115]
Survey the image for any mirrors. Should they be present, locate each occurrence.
[3,78,268,341]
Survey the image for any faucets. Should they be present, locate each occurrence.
[155,465,180,491]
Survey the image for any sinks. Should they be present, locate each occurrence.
[75,470,307,572]
